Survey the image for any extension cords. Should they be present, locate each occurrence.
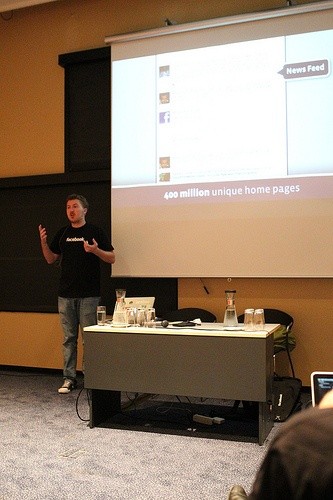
[193,414,214,426]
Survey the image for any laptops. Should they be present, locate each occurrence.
[310,371,333,407]
[113,297,155,323]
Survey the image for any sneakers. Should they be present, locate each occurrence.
[228,485,247,500]
[58,378,77,394]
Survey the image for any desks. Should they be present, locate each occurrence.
[83,321,280,445]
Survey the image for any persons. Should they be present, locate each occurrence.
[39,193,115,393]
[228,386,333,500]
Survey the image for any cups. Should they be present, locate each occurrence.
[137,305,146,328]
[125,307,136,329]
[244,308,265,331]
[96,306,107,325]
[146,308,155,328]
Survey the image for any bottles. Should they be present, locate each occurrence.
[112,289,127,328]
[222,290,239,327]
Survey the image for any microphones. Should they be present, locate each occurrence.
[140,320,168,328]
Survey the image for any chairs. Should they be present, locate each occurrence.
[237,309,297,380]
[169,308,216,325]
